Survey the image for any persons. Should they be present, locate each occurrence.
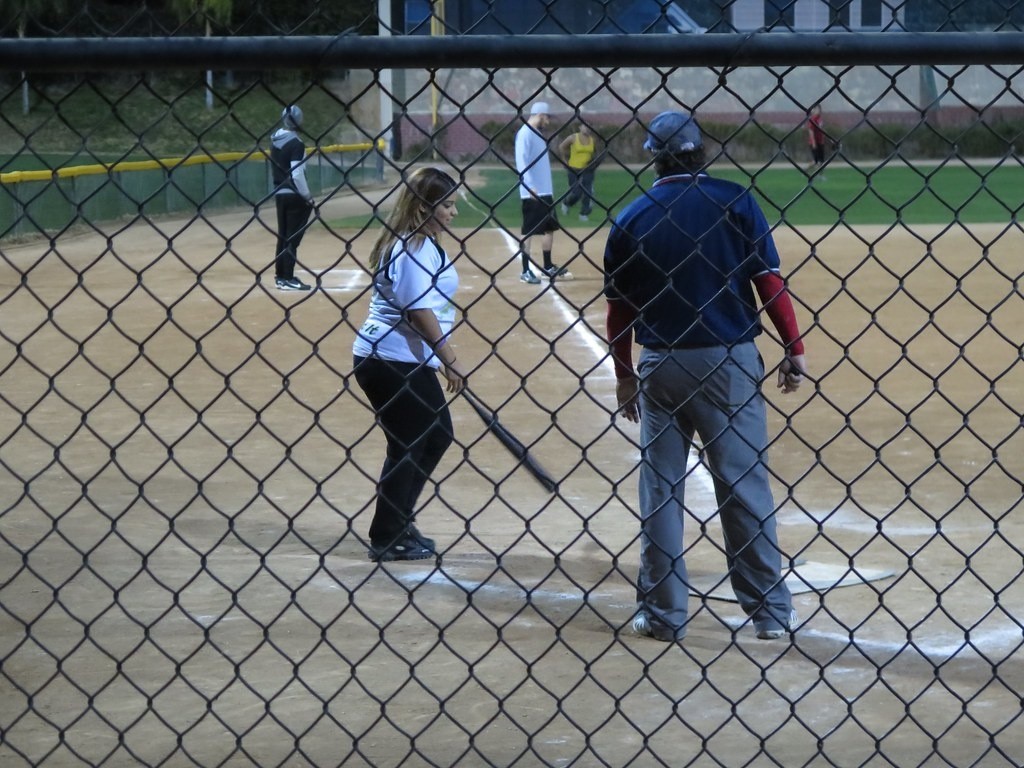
[808,105,826,181]
[270,105,312,290]
[559,120,600,220]
[515,102,573,283]
[604,102,805,639]
[352,168,467,560]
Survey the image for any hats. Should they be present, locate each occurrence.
[283,106,302,126]
[643,111,702,154]
[531,102,551,114]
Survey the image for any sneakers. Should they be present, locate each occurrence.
[633,609,687,640]
[541,264,573,280]
[275,276,283,288]
[281,277,311,290]
[756,606,795,639]
[402,526,436,551]
[519,270,541,283]
[367,533,433,561]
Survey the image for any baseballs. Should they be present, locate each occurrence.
[563,272,573,280]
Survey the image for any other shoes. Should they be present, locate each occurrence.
[561,202,568,212]
[579,214,588,221]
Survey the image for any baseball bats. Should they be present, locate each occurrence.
[459,389,559,494]
